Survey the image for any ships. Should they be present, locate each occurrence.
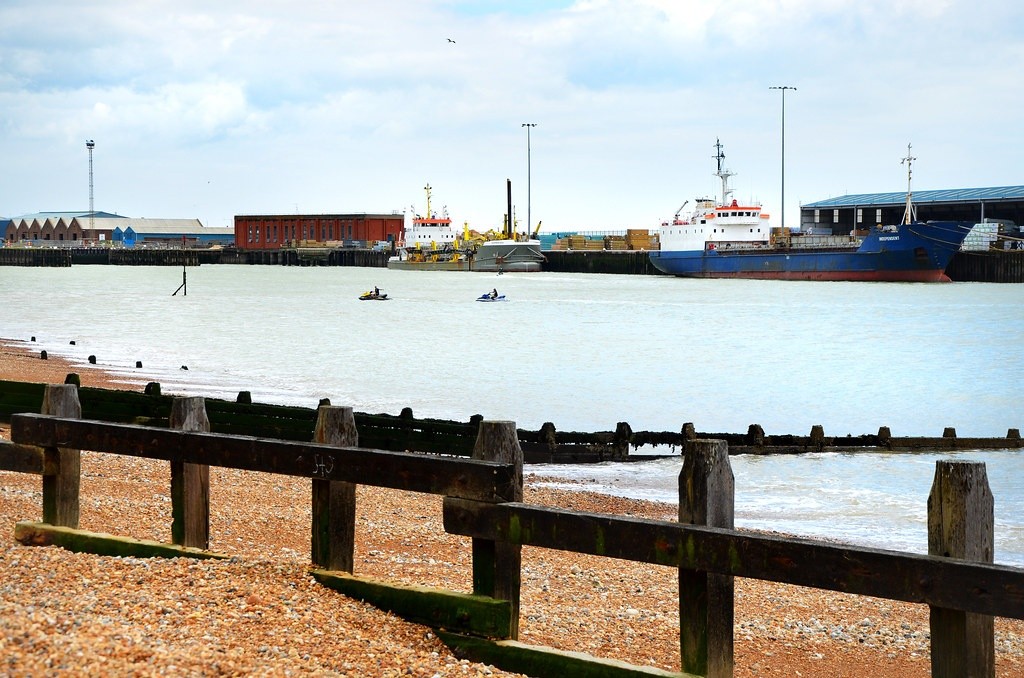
[384,179,544,271]
[646,135,975,283]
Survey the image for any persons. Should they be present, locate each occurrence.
[489,287,499,301]
[374,285,380,296]
[628,243,634,250]
